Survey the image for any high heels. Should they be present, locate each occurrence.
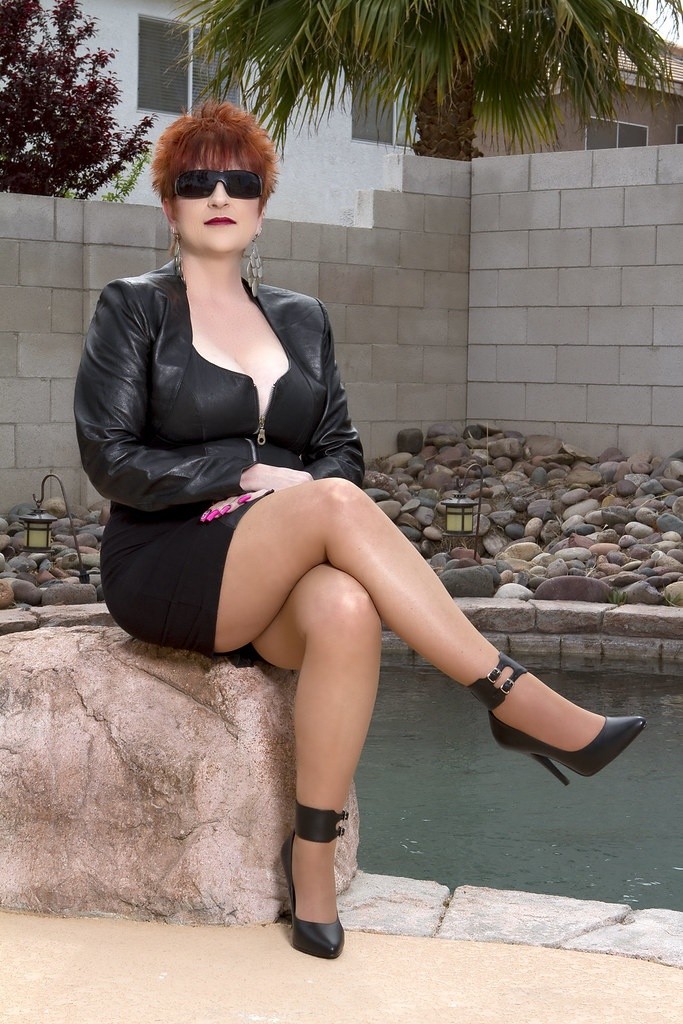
[282,798,349,959]
[468,651,648,785]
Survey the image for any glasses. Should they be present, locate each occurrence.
[172,169,265,199]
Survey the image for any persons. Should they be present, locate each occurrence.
[73,98,648,961]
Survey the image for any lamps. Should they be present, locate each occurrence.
[440,483,479,534]
[19,500,57,553]
